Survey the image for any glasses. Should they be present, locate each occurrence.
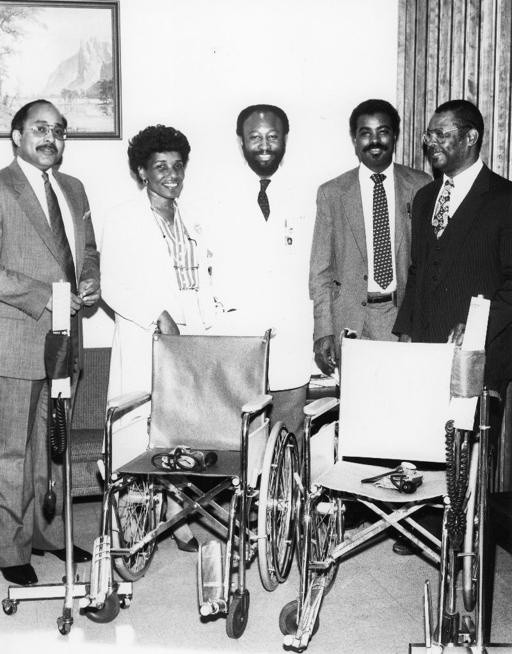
[422,128,458,146]
[21,125,67,140]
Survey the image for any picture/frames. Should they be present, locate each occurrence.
[0,0,123,141]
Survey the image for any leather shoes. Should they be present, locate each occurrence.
[393,525,435,554]
[171,529,200,553]
[0,564,37,584]
[31,545,93,562]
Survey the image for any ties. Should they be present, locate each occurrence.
[257,179,271,222]
[432,178,454,239]
[370,174,392,290]
[41,172,76,292]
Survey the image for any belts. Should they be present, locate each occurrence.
[365,295,391,304]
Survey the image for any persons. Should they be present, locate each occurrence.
[0,100,105,585]
[309,98,434,383]
[391,98,512,556]
[201,106,315,472]
[98,125,215,553]
